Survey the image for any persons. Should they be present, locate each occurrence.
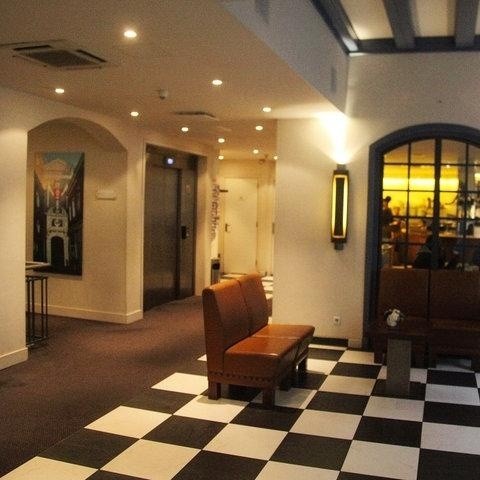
[381,196,435,268]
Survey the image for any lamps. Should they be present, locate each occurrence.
[331,164,349,250]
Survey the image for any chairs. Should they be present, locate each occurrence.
[238,273,315,388]
[202,279,299,410]
[428,269,480,373]
[374,267,428,369]
